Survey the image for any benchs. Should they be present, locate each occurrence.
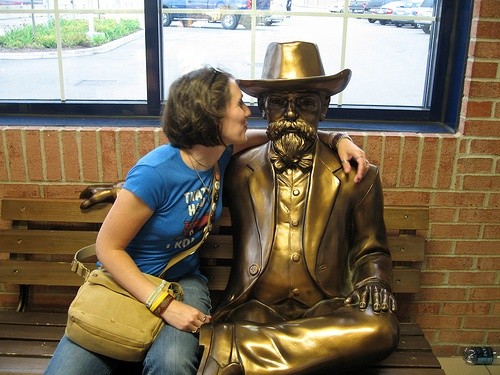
[0,198,445,375]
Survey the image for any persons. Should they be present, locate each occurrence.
[42,63,370,375]
[286,0,293,18]
[77,40,401,375]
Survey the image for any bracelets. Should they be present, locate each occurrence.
[328,131,354,150]
[145,288,174,316]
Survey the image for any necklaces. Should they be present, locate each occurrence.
[184,147,214,202]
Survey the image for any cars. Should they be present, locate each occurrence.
[328,0,435,34]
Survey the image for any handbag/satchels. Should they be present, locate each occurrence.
[65,268,182,360]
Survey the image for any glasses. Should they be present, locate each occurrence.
[204,65,224,99]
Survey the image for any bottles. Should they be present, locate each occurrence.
[463,346,500,365]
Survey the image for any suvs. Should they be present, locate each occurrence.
[160,0,286,31]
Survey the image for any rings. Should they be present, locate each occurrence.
[201,316,207,322]
[356,154,364,163]
[191,327,197,333]
[198,324,204,330]
[365,159,369,163]
[340,159,349,164]
